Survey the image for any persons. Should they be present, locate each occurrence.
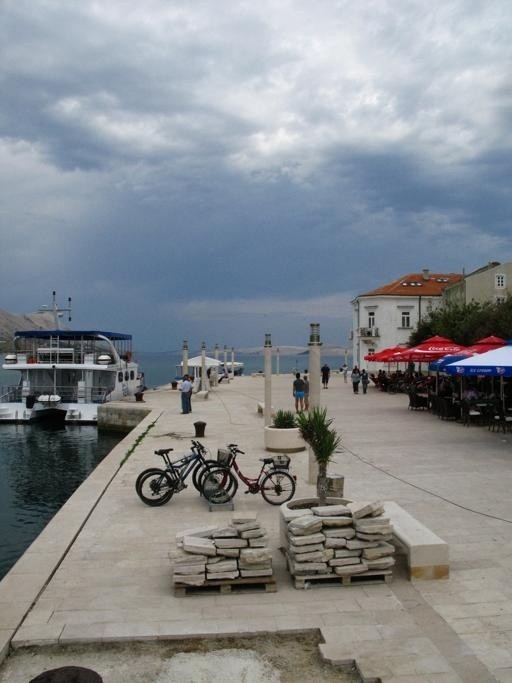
[301,374,310,412]
[320,362,331,389]
[217,366,229,383]
[291,372,306,413]
[189,373,194,413]
[352,365,512,419]
[341,362,348,384]
[177,376,194,414]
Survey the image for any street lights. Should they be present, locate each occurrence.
[231,345,234,378]
[223,344,228,378]
[307,321,321,486]
[344,348,348,366]
[201,340,207,391]
[264,332,273,425]
[276,347,279,375]
[214,343,219,385]
[182,336,189,377]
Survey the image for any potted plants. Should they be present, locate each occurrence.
[262,408,308,454]
[278,402,355,548]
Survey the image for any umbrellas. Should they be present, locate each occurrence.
[180,354,221,378]
[363,334,512,376]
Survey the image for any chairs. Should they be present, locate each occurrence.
[369,370,512,436]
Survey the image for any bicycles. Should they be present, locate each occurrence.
[136,438,296,507]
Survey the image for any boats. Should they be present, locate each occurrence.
[174,354,245,382]
[0,290,143,423]
